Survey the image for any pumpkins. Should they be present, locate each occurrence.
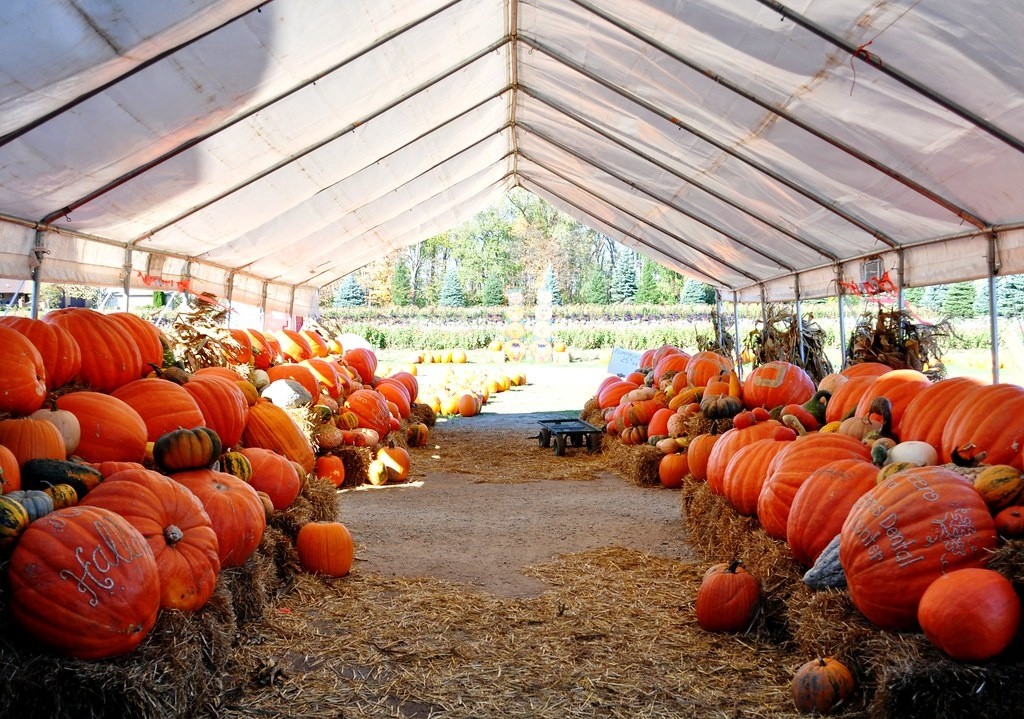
[2,302,1024,712]
